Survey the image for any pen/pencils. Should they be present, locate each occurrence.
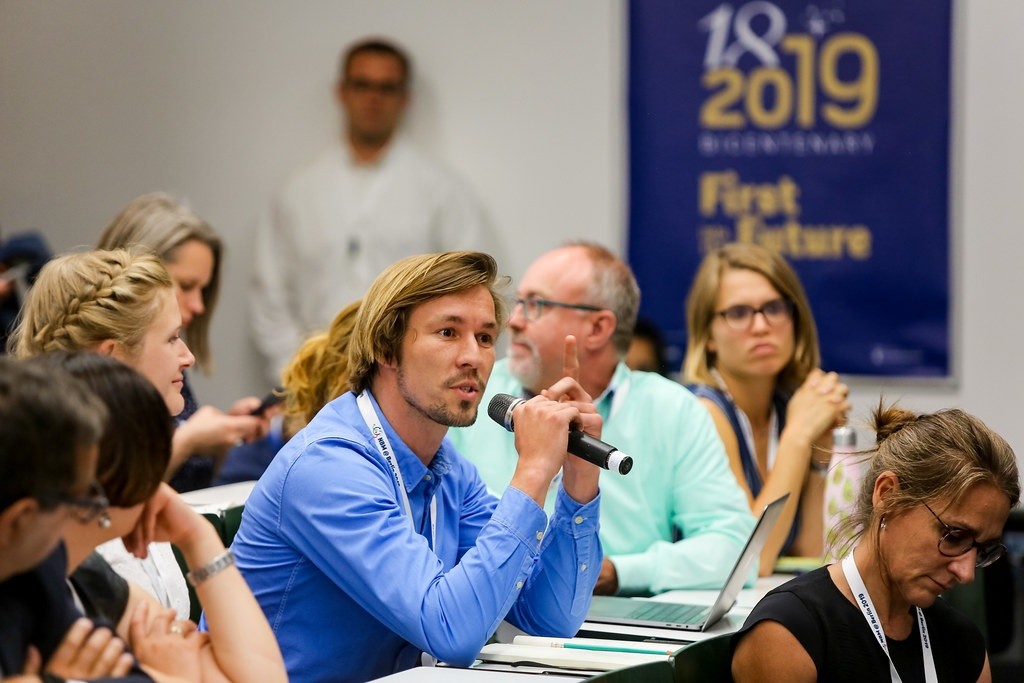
[551,642,675,656]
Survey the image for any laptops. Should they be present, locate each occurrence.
[582,491,792,632]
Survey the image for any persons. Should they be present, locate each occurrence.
[247,36,478,394]
[682,241,852,578]
[729,398,1021,683]
[0,192,761,683]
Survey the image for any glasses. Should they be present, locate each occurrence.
[504,295,600,324]
[707,300,792,330]
[52,475,109,525]
[899,476,1009,568]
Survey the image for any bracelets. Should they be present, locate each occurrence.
[809,462,829,471]
[186,551,233,587]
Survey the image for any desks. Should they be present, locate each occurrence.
[363,569,797,683]
[178,476,258,514]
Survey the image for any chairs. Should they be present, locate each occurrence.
[169,504,244,623]
[583,631,739,682]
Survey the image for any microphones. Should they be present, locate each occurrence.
[488,394,633,475]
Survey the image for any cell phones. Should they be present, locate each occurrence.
[249,386,287,417]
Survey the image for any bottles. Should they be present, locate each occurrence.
[823,428,863,566]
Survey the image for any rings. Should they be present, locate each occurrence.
[171,626,182,634]
